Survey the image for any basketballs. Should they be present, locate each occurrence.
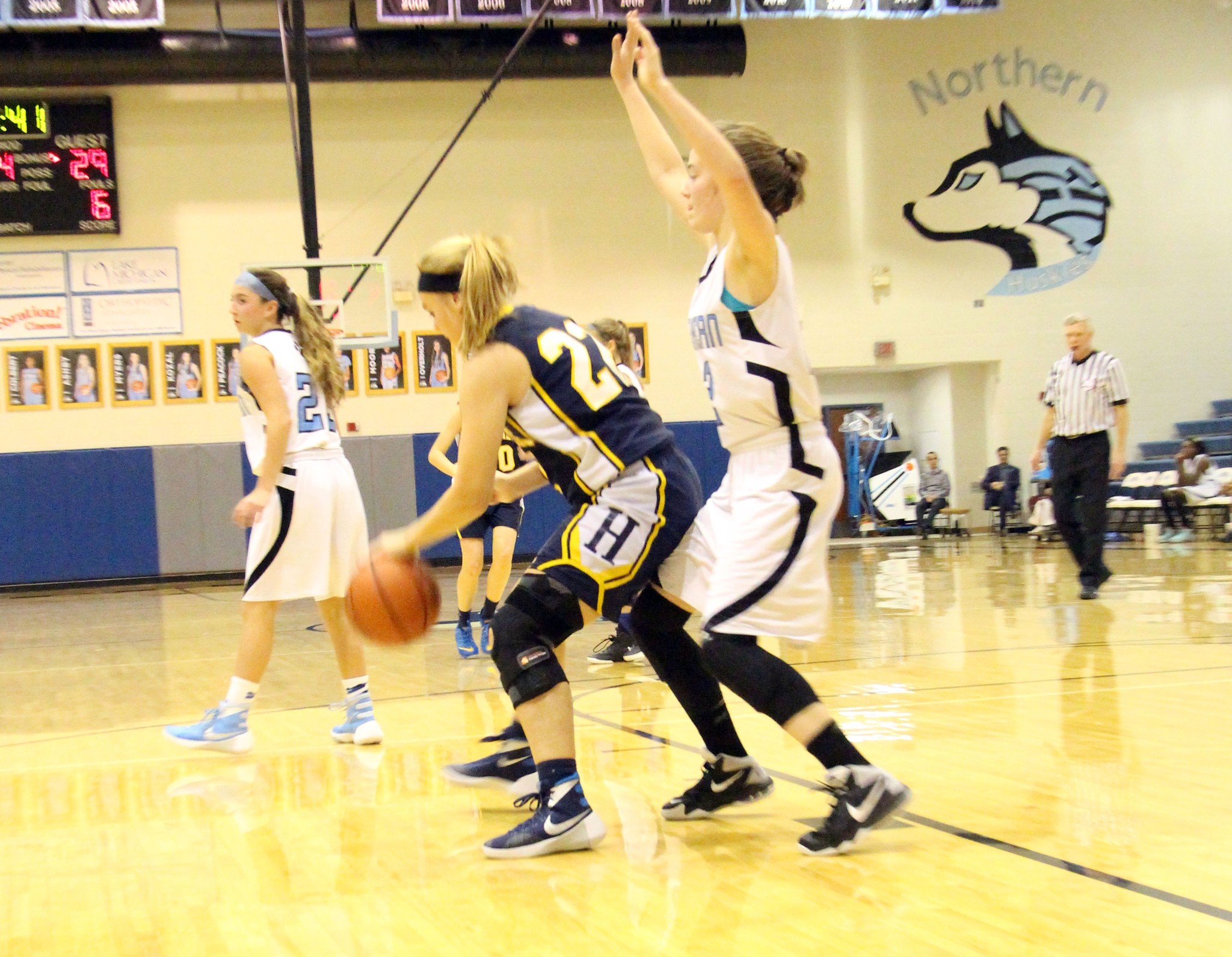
[345,556,441,647]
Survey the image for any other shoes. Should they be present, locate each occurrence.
[1027,526,1052,534]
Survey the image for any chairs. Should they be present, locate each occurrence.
[1034,467,1232,541]
[988,490,1023,533]
[919,492,952,538]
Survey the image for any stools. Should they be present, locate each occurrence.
[939,508,971,538]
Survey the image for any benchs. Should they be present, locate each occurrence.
[1123,397,1232,474]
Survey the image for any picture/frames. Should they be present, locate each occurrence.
[110,341,155,407]
[411,330,459,393]
[209,339,245,404]
[0,249,66,297]
[71,290,182,336]
[162,339,207,403]
[0,297,68,340]
[68,245,181,294]
[365,332,407,396]
[57,344,103,408]
[5,344,51,410]
[331,333,359,396]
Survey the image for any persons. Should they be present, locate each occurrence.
[164,270,382,752]
[1026,440,1056,541]
[1031,314,1130,600]
[609,11,911,859]
[916,452,950,540]
[979,446,1020,537]
[429,409,530,654]
[585,318,645,666]
[372,235,773,854]
[1156,437,1221,543]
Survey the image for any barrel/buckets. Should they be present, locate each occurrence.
[1224,522,1232,532]
[1142,523,1163,542]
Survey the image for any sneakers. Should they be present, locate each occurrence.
[1157,532,1170,543]
[484,771,606,859]
[455,620,477,658]
[1167,527,1195,543]
[795,766,913,856]
[330,695,384,745]
[663,755,775,823]
[1081,585,1099,599]
[442,743,541,797]
[480,718,527,743]
[586,629,645,664]
[478,608,495,652]
[163,703,253,752]
